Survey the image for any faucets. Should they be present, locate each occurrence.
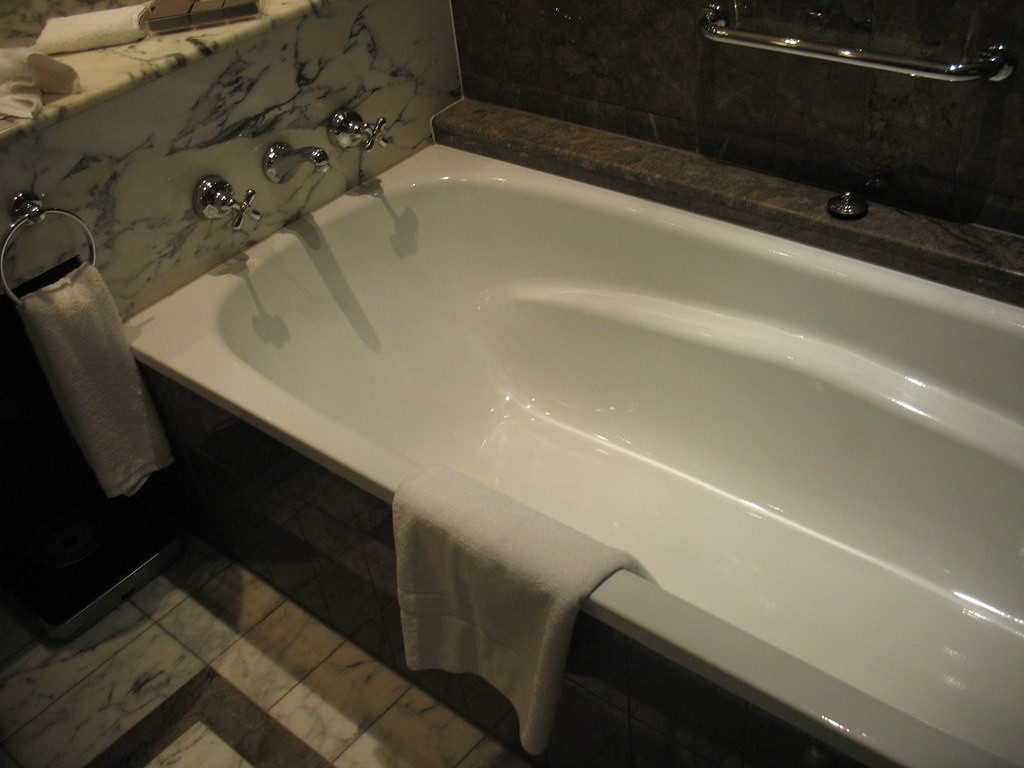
[279,145,331,176]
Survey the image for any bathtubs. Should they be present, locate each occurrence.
[124,142,1024,768]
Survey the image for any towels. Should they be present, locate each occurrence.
[0,45,82,122]
[17,259,176,503]
[391,457,657,756]
[35,0,159,54]
